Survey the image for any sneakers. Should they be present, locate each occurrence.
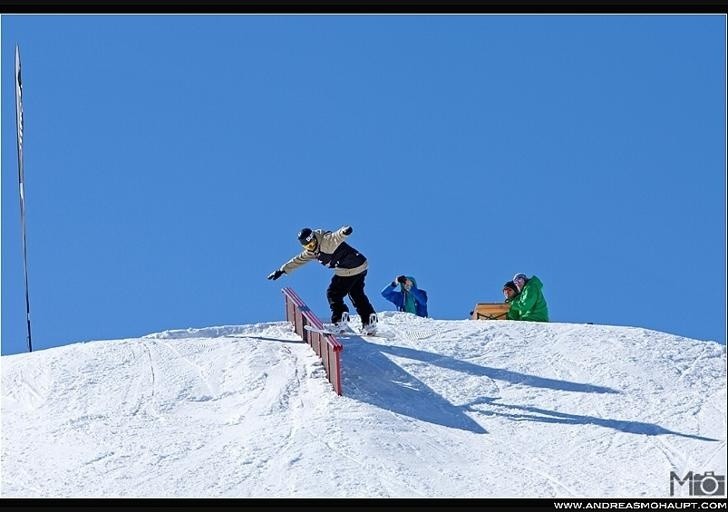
[362,324,376,331]
[327,320,346,332]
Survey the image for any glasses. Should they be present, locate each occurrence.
[304,239,316,249]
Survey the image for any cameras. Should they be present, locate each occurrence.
[397,276,406,283]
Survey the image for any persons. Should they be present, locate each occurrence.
[266,225,378,335]
[501,281,518,302]
[504,272,550,322]
[380,274,428,318]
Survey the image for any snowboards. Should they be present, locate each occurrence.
[304,325,395,338]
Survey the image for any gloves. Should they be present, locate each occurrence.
[267,269,285,280]
[343,226,353,235]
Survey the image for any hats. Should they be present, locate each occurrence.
[513,273,528,281]
[503,281,519,293]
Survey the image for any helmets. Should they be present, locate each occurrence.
[297,228,314,246]
